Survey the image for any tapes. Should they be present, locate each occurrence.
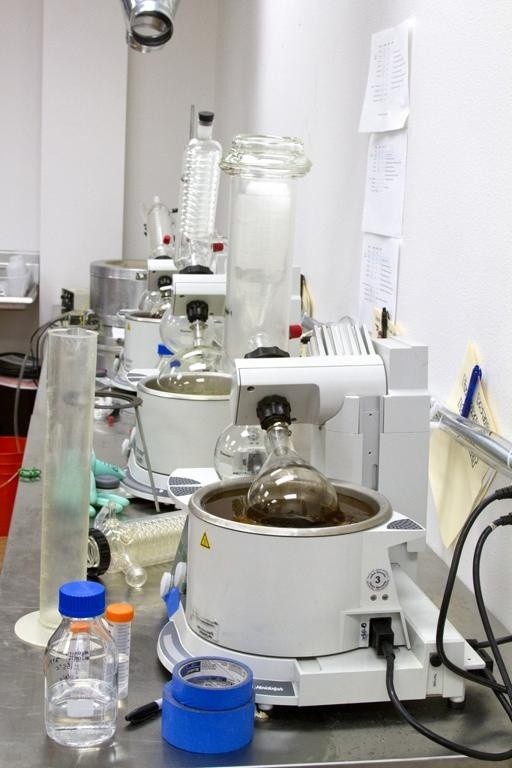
[161,656,254,753]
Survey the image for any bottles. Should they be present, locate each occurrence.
[220,134,313,358]
[157,300,240,397]
[146,110,222,272]
[249,394,342,531]
[44,579,134,750]
[93,366,113,419]
[39,329,97,634]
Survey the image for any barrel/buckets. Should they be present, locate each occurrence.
[1,435,27,534]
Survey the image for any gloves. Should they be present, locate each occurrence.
[89,449,130,518]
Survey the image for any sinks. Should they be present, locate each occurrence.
[0,253,39,310]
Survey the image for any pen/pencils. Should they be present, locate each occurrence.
[125,699,161,724]
[464,365,482,421]
